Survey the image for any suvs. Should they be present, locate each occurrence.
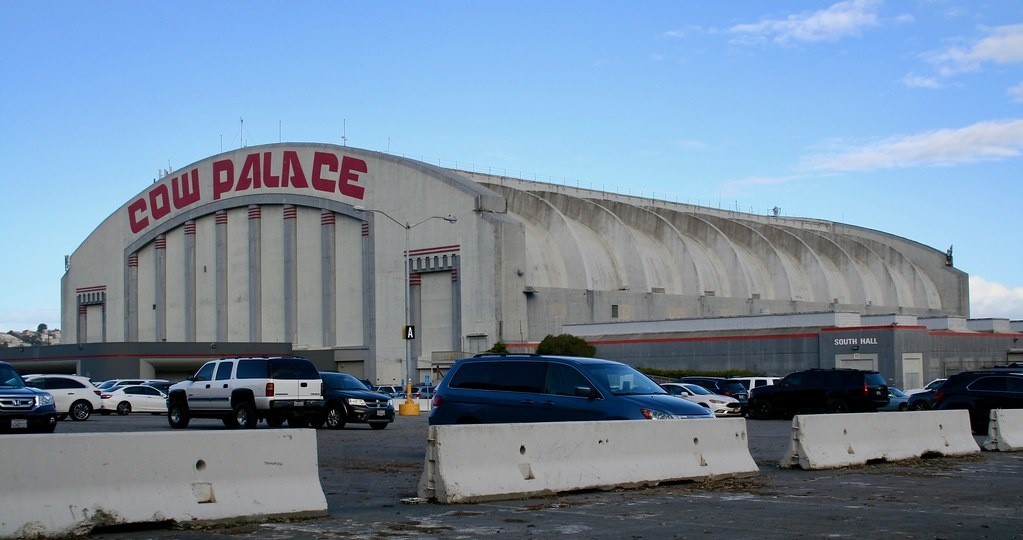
[747,368,891,419]
[166,355,326,429]
[429,353,716,421]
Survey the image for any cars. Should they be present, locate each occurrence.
[658,383,742,417]
[359,379,436,399]
[0,361,57,434]
[671,374,949,412]
[100,384,168,416]
[319,372,396,429]
[24,374,102,421]
[96,378,170,390]
[929,367,1023,435]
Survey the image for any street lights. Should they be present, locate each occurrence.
[353,205,458,398]
